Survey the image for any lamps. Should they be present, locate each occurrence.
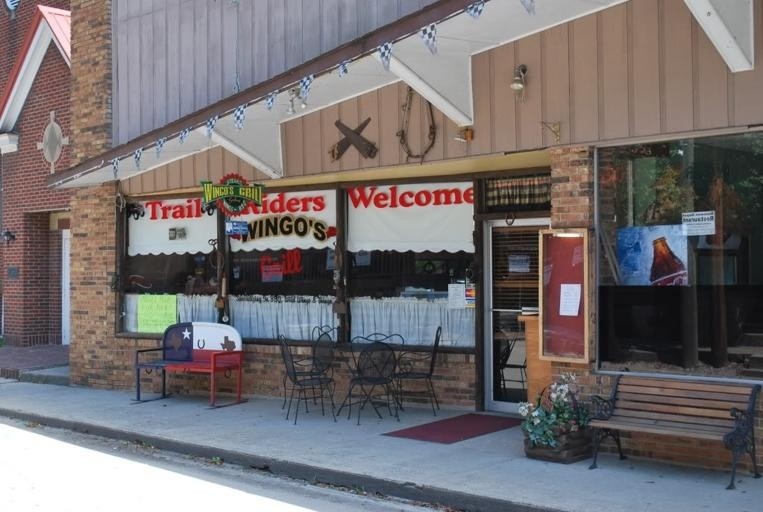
[509,63,527,91]
[1,227,16,244]
[453,128,473,142]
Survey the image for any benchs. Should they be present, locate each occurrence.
[132,321,245,407]
[581,373,759,491]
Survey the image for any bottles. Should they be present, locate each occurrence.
[648,234,688,286]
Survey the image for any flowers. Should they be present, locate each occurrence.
[519,370,593,450]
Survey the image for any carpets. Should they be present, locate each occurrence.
[379,414,527,445]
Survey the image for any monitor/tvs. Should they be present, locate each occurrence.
[506,253,533,273]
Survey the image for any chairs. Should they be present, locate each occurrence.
[276,333,338,426]
[281,325,341,412]
[493,322,526,402]
[333,326,442,429]
